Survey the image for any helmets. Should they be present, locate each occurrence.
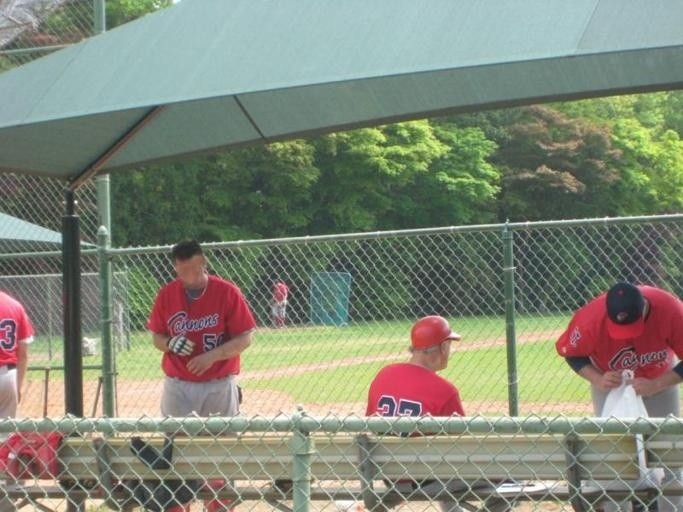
[411,314,462,350]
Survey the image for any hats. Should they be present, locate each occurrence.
[604,282,648,343]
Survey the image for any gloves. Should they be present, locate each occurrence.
[166,335,196,358]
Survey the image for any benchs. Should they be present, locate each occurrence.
[0,428,683,512]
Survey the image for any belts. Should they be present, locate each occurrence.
[7,364,17,370]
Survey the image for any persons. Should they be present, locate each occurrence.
[269,278,289,328]
[362,314,514,512]
[551,279,682,512]
[143,238,257,511]
[0,290,35,444]
